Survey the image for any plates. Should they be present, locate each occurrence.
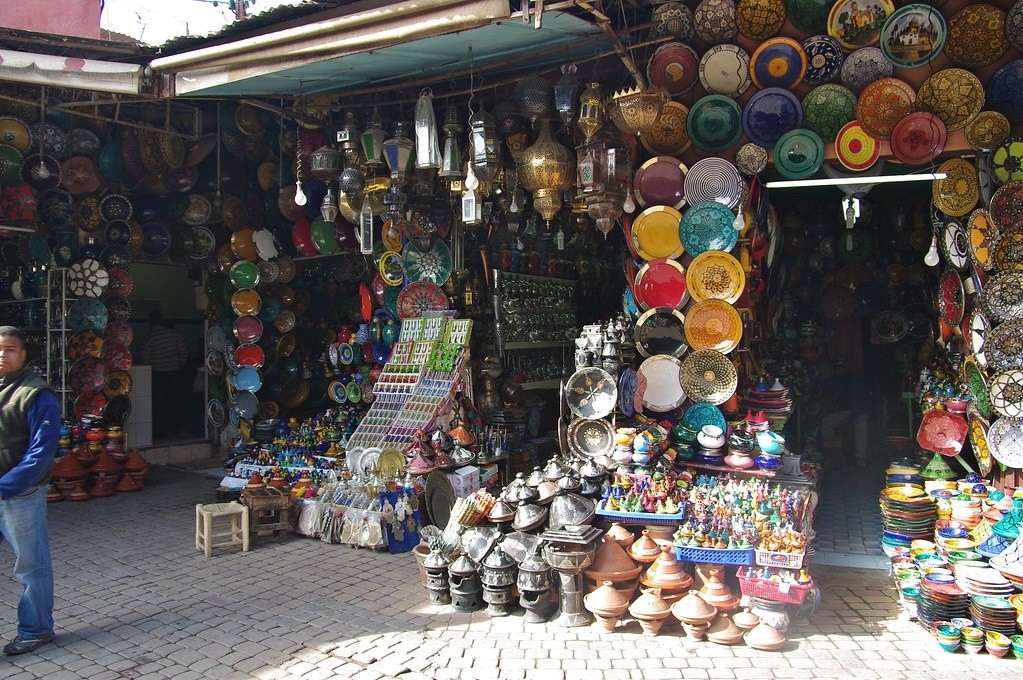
[558,1,1023,558]
[424,469,456,531]
[346,447,405,478]
[0,112,454,434]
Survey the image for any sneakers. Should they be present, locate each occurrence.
[3,629,55,654]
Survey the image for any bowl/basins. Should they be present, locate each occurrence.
[880,478,1023,662]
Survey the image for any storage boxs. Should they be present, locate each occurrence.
[446,463,499,502]
[215,486,243,505]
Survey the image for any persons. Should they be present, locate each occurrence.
[0,325,61,655]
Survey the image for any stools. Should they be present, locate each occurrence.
[195,487,291,558]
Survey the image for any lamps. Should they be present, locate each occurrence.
[310,36,636,241]
